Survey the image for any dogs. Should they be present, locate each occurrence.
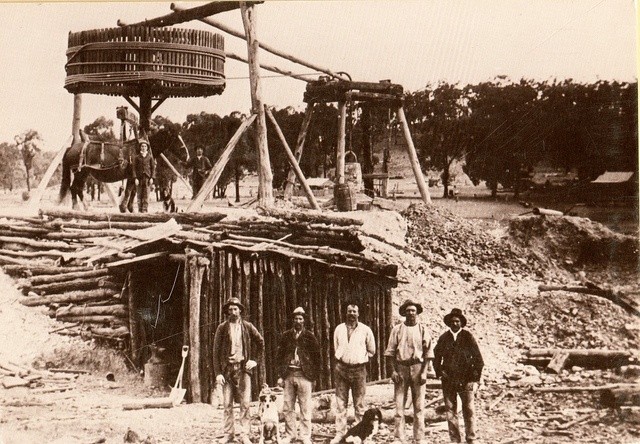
[258,394,281,444]
[336,408,383,444]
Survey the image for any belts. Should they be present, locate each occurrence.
[288,367,301,371]
[338,362,368,368]
[398,359,420,366]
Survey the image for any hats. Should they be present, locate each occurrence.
[443,308,467,327]
[223,297,245,315]
[194,143,206,152]
[291,306,309,319]
[138,139,150,151]
[399,299,423,316]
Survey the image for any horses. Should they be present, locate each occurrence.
[58,123,194,214]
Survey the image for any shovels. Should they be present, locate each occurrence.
[169,345,189,406]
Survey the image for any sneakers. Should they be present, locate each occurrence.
[281,437,291,443]
[242,436,252,444]
[301,437,312,444]
[390,440,404,443]
[220,435,233,444]
[447,440,458,444]
[329,435,342,443]
[468,439,480,444]
[414,440,427,444]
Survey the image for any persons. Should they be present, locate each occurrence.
[178,143,213,200]
[330,305,376,444]
[433,308,485,444]
[132,139,155,213]
[384,300,434,444]
[274,307,322,444]
[212,297,265,444]
